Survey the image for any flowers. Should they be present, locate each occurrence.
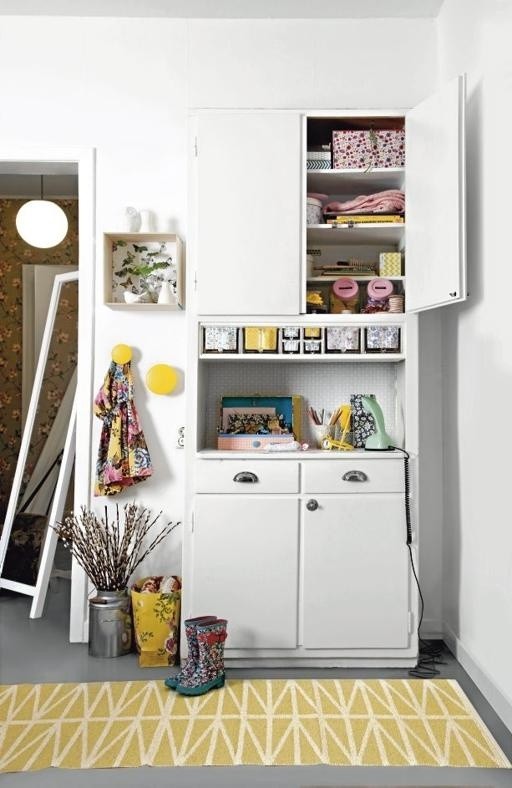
[48,497,182,591]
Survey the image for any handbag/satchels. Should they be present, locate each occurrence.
[132,576,181,667]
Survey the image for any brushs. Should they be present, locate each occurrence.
[313,258,366,269]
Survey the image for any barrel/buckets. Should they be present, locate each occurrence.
[85,587,131,659]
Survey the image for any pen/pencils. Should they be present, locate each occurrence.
[306,407,343,425]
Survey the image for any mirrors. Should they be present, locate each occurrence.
[0,270,78,620]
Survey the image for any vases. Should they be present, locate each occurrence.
[98,591,132,656]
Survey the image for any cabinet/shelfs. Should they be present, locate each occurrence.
[198,324,409,455]
[195,73,466,322]
[195,460,412,651]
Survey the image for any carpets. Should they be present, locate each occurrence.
[1,678,511,773]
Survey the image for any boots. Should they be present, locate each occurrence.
[165,616,226,695]
